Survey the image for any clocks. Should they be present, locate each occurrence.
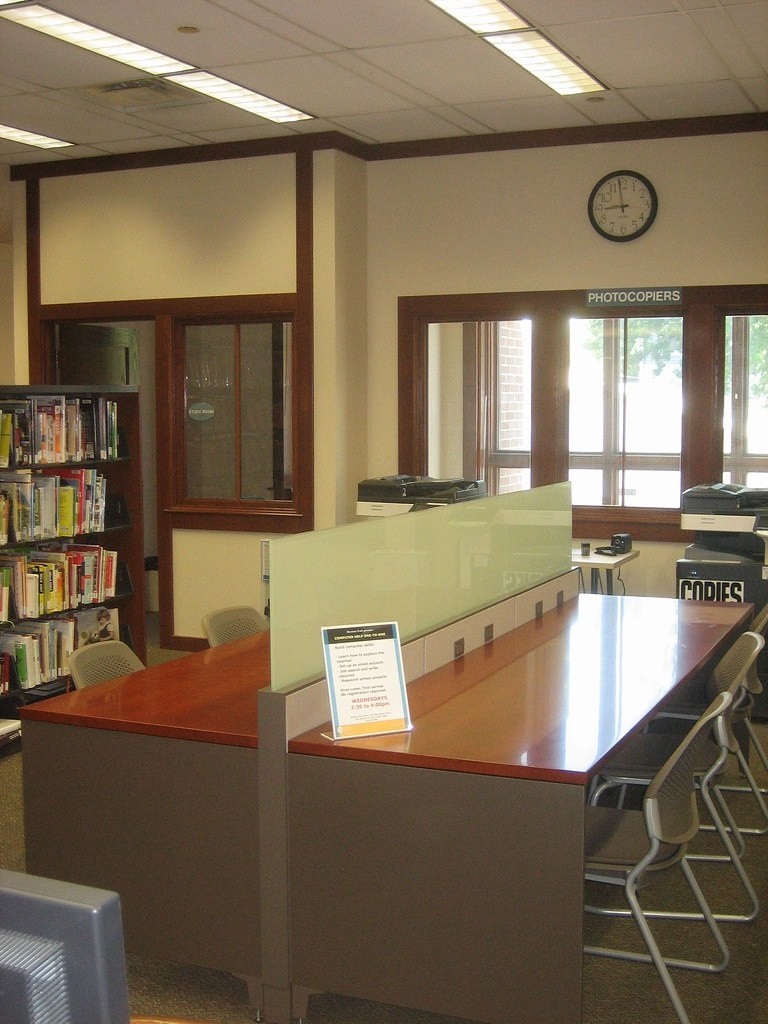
[588,170,658,242]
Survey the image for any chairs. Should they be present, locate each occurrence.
[583,603,768,1024]
[202,605,269,648]
[68,640,145,690]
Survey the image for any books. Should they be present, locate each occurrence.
[0,468,106,546]
[0,543,118,622]
[0,607,120,696]
[0,718,22,745]
[0,393,119,468]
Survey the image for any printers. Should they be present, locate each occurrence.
[356,475,488,521]
[676,483,768,722]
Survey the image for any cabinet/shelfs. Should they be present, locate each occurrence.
[0,385,147,700]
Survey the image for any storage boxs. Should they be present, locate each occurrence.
[0,719,22,759]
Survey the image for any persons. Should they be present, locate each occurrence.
[84,610,114,646]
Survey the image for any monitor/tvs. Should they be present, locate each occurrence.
[0,868,130,1024]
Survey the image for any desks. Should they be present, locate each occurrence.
[286,592,754,1024]
[572,548,639,595]
[18,630,270,1023]
